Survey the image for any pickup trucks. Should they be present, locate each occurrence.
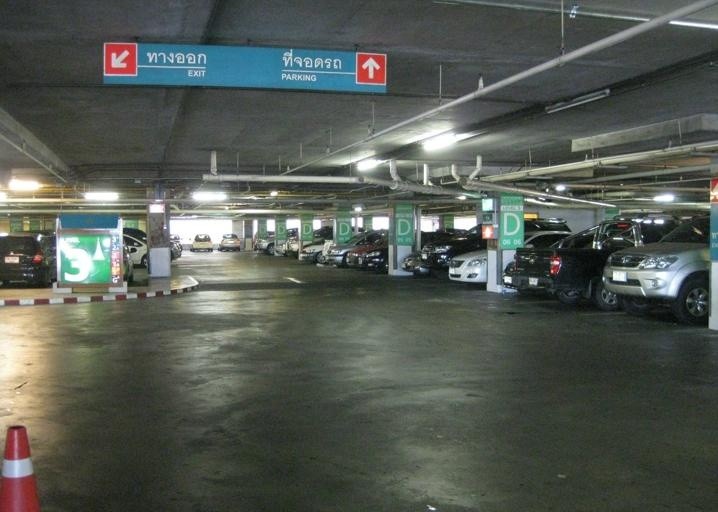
[511,209,686,313]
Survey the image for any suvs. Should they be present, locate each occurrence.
[1,229,57,288]
[600,212,710,330]
[419,216,573,281]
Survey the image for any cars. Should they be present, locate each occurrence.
[122,226,183,269]
[253,227,470,277]
[190,233,214,252]
[123,238,136,282]
[447,230,574,283]
[217,233,242,252]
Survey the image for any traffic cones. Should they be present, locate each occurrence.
[0,421,46,512]
